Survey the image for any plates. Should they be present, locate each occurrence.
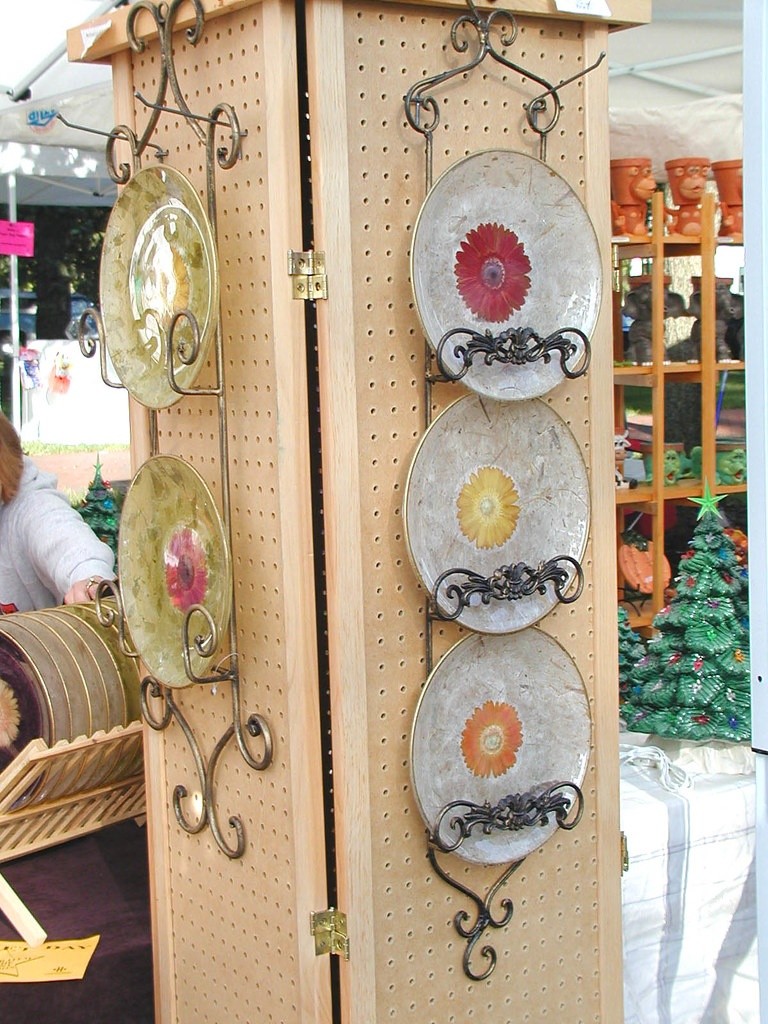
[409,625,592,865]
[117,455,233,690]
[99,163,219,410]
[402,391,591,635]
[410,149,603,400]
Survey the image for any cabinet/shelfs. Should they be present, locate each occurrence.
[612,191,749,638]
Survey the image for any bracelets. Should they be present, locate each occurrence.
[86,577,100,600]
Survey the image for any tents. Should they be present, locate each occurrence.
[0,0,744,436]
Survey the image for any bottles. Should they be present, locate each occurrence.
[49,359,70,394]
[65,291,95,340]
[18,351,43,390]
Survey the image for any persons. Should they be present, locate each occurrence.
[3,330,27,400]
[0,408,118,618]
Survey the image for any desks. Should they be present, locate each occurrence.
[618,730,761,1024]
[1,816,157,1024]
